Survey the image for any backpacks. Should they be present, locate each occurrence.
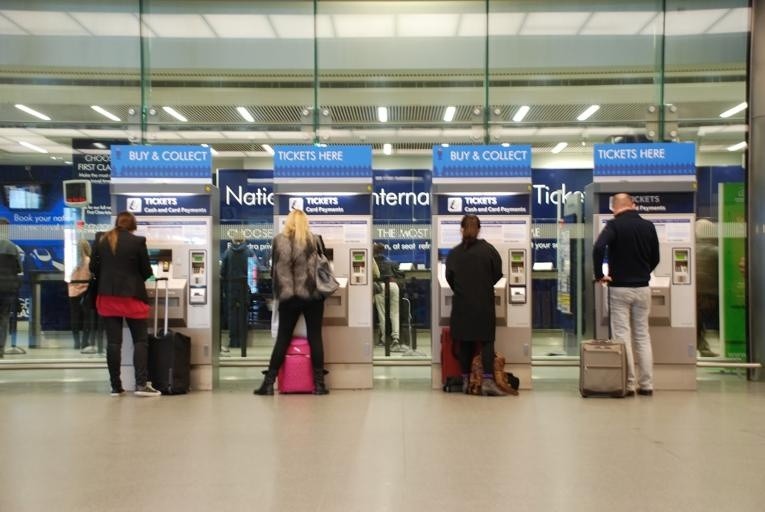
[469,350,520,396]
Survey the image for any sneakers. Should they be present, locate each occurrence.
[110,387,126,396]
[390,338,399,347]
[701,349,721,358]
[624,390,635,397]
[134,382,161,397]
[636,389,653,396]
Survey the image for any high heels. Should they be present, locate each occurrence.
[481,377,508,397]
[462,375,468,393]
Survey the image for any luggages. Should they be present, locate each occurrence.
[580,280,627,398]
[147,277,191,395]
[276,336,317,396]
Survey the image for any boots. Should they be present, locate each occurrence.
[253,370,276,395]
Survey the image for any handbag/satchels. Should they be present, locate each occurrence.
[315,234,340,297]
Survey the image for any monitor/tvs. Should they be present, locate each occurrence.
[62,179,92,207]
[0,182,47,211]
[145,257,160,279]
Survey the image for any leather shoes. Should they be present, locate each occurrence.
[316,369,330,395]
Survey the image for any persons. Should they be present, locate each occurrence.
[221,232,260,347]
[0,215,23,359]
[254,208,339,395]
[93,212,162,397]
[89,233,109,353]
[593,193,660,396]
[372,243,406,351]
[373,256,380,304]
[68,240,94,352]
[694,219,718,358]
[446,214,507,396]
